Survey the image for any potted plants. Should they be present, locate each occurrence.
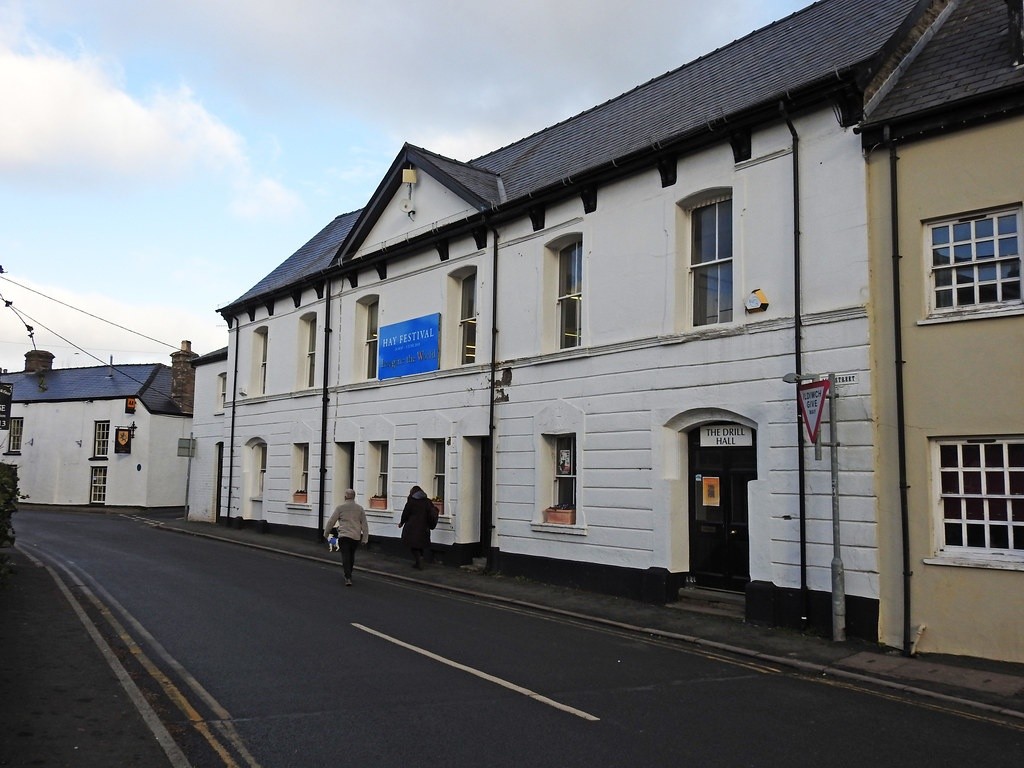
[545,504,576,525]
[431,496,444,515]
[292,490,307,503]
[370,495,387,509]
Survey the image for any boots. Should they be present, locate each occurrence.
[415,551,424,570]
[412,562,418,569]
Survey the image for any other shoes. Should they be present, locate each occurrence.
[345,577,353,586]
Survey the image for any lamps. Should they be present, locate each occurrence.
[114,420,137,455]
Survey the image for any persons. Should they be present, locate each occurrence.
[322,489,369,588]
[398,486,435,572]
